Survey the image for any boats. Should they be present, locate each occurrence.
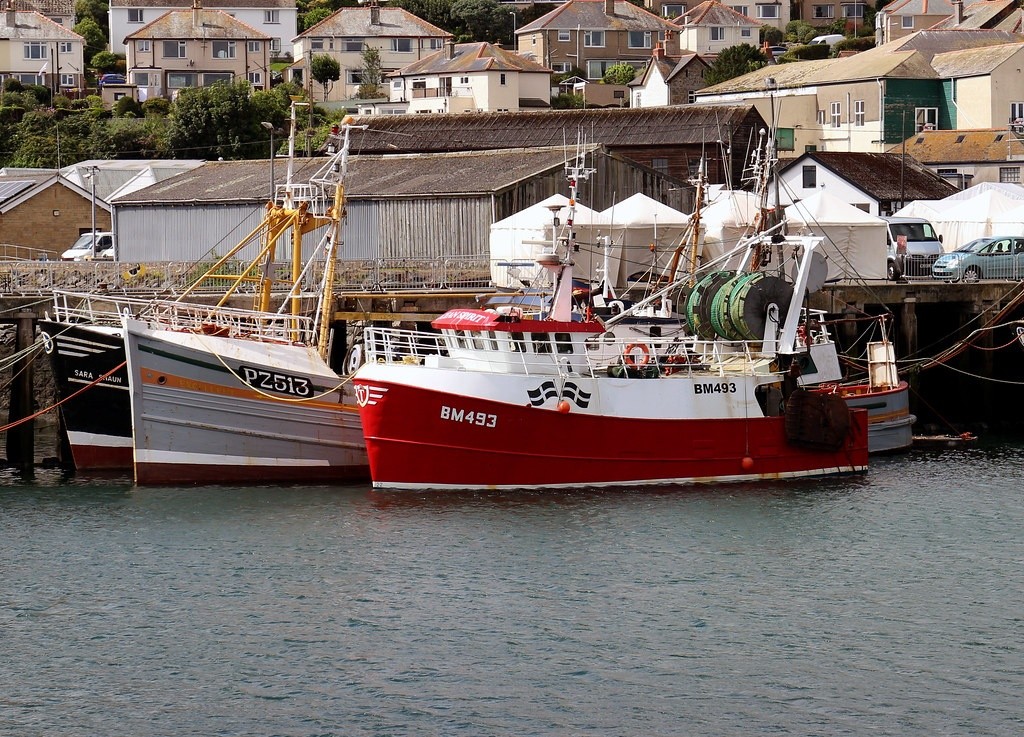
[38,94,917,490]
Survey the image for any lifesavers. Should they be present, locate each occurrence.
[624,343,649,370]
[804,336,812,345]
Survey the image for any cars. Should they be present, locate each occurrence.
[768,41,800,57]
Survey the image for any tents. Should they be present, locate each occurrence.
[601,192,706,289]
[893,183,1024,254]
[490,194,626,290]
[784,191,888,279]
[691,196,803,281]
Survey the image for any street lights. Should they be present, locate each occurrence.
[509,11,516,55]
[260,122,284,202]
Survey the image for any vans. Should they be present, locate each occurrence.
[807,34,845,54]
[875,216,945,281]
[932,236,1024,283]
[61,232,114,261]
